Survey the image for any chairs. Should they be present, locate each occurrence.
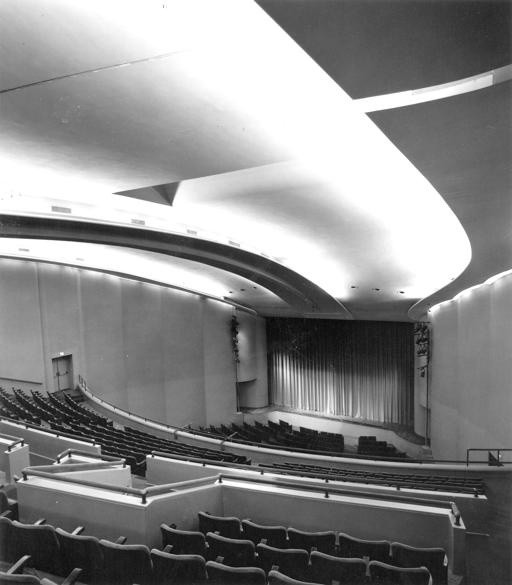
[0,386,488,585]
